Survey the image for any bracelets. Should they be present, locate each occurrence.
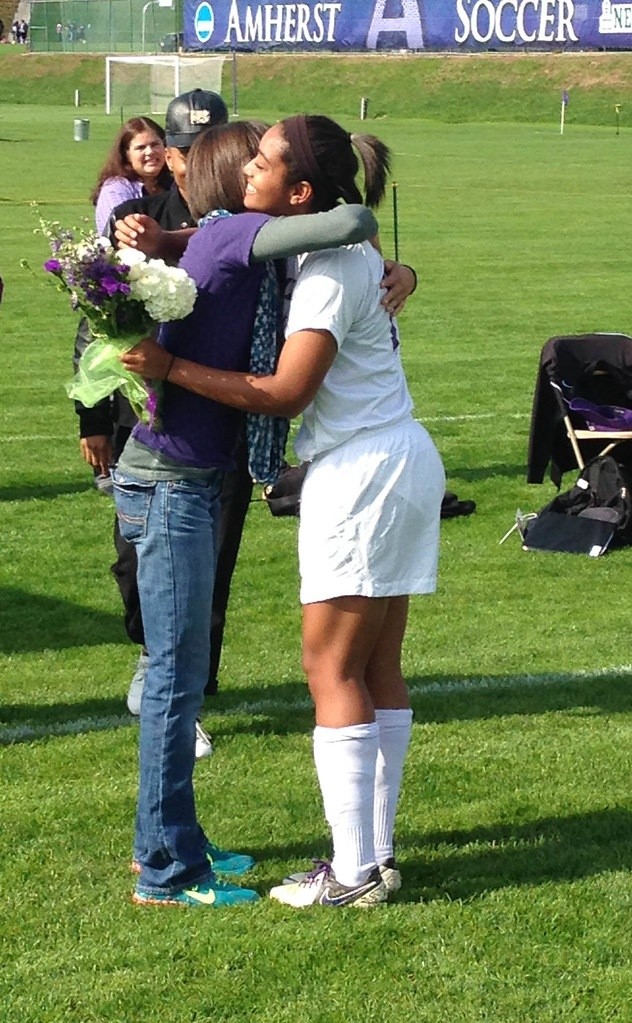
[402,264,417,295]
[164,355,176,378]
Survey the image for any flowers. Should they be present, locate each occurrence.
[18,197,198,432]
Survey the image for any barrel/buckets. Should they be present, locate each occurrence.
[74,118,90,141]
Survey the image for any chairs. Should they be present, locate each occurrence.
[542,330,632,468]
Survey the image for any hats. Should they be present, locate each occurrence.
[163,87,227,152]
[568,396,632,431]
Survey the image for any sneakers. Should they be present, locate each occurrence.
[124,651,150,715]
[269,863,388,913]
[279,858,403,894]
[129,837,256,878]
[133,877,261,909]
[194,722,214,761]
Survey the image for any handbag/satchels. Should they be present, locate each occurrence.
[266,465,304,516]
[519,454,632,556]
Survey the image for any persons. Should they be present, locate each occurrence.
[95,117,165,265]
[56,20,86,42]
[11,19,28,44]
[121,116,447,910]
[73,87,253,758]
[108,122,418,909]
